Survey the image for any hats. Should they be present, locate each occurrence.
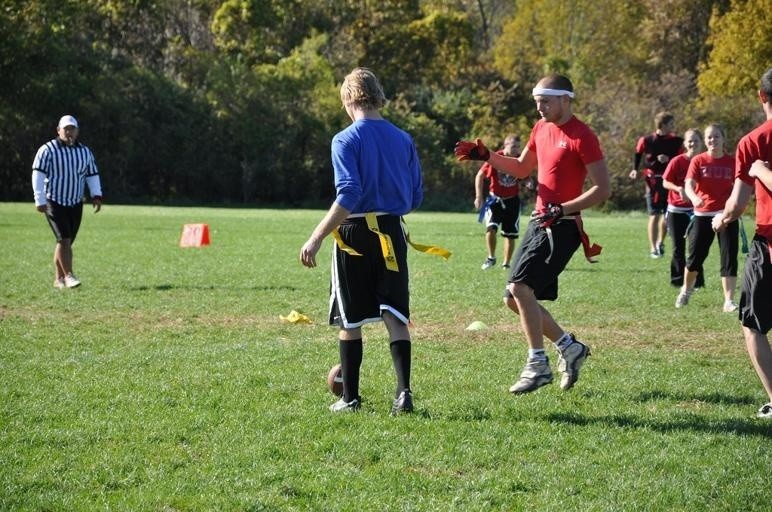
[58,115,78,129]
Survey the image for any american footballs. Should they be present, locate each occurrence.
[328,364,344,396]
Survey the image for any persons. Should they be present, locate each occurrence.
[675,124,740,313]
[662,128,706,291]
[300,68,425,417]
[628,113,686,258]
[711,68,772,418]
[454,72,609,397]
[749,157,772,192]
[32,115,102,289]
[474,132,534,270]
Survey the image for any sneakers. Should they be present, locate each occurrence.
[722,301,740,313]
[53,276,65,288]
[481,256,496,271]
[507,356,554,394]
[756,401,772,420]
[651,241,664,260]
[557,333,591,391]
[675,286,693,309]
[64,273,80,288]
[328,395,362,415]
[390,388,414,417]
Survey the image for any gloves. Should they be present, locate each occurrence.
[529,202,566,229]
[453,136,490,164]
